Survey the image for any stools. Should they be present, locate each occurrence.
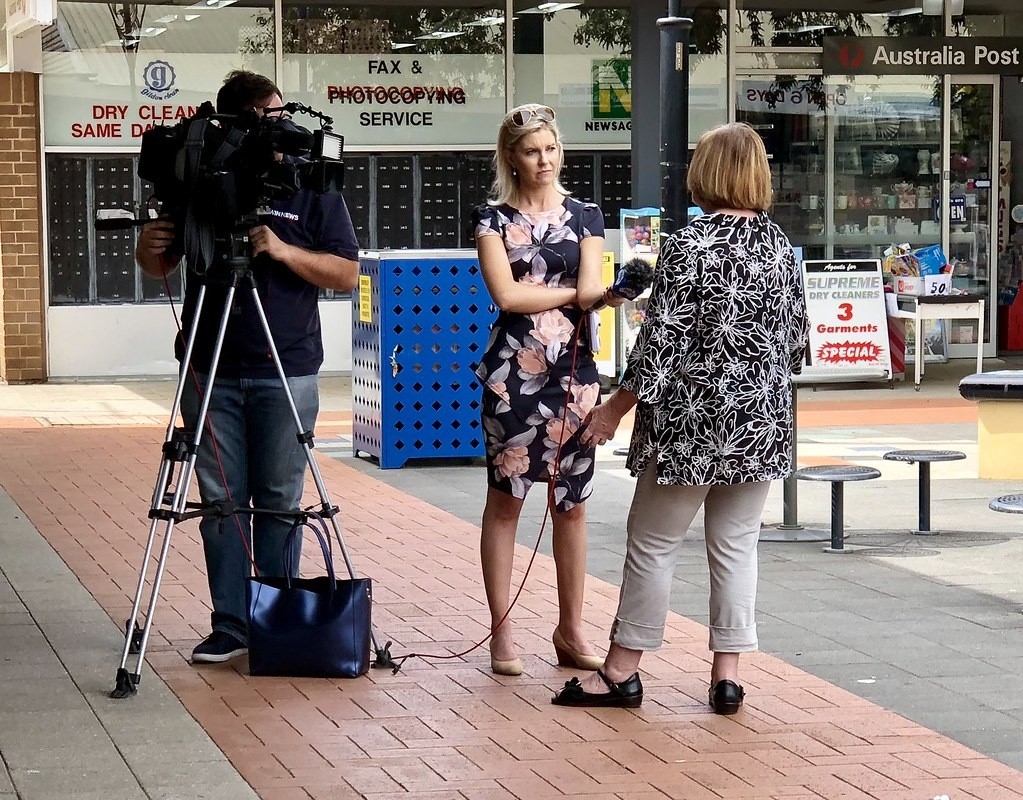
[884,449,966,536]
[793,464,881,554]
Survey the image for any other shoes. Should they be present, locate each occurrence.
[551,668,642,707]
[192,630,249,662]
[709,678,744,714]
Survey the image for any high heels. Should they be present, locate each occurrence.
[489,640,522,675]
[552,624,606,670]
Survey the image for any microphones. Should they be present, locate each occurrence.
[583,258,653,316]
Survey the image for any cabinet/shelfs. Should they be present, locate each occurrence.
[793,136,996,358]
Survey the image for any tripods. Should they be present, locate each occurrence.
[95,218,398,698]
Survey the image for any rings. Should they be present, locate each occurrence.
[600,441,606,442]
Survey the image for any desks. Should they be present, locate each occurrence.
[756,367,888,542]
[887,292,987,391]
[958,369,1023,483]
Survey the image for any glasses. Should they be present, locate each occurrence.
[503,107,556,138]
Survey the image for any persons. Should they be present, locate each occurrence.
[135,71,360,663]
[473,103,624,675]
[552,122,811,714]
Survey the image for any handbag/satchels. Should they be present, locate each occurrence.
[246,512,372,679]
